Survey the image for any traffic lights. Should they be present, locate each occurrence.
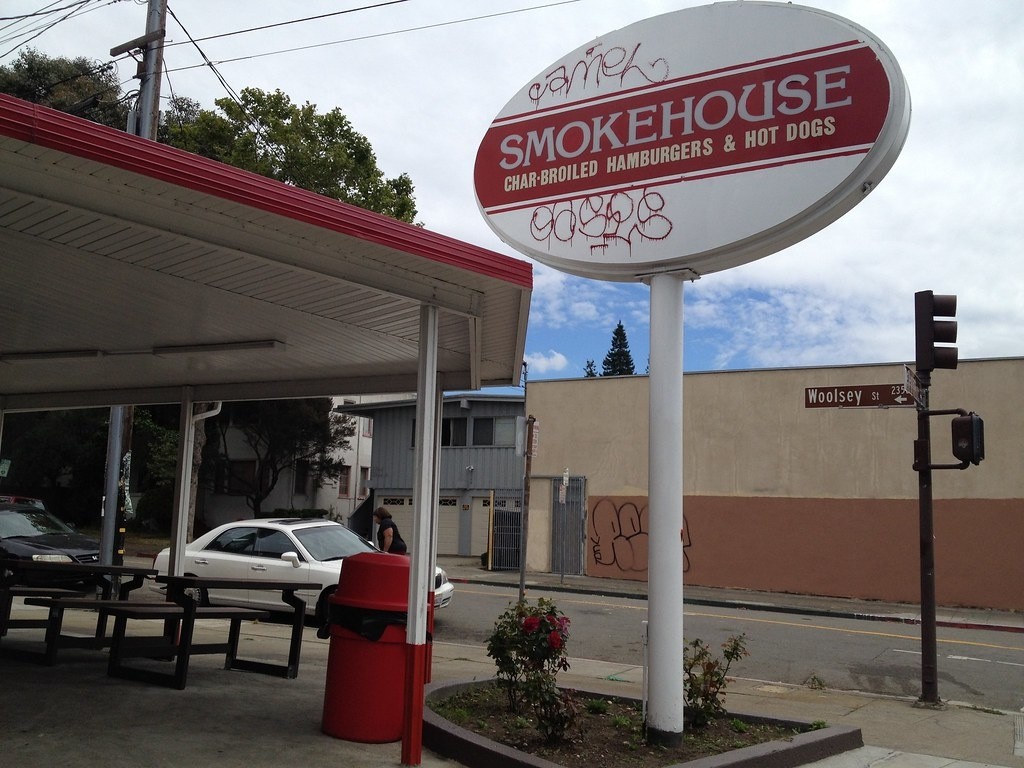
[915,290,959,371]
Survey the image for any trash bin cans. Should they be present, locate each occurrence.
[321,551,411,744]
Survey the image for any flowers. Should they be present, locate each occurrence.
[524,605,571,651]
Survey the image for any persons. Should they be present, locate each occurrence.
[373,507,408,555]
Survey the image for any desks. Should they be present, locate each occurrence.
[0,557,159,649]
[108,575,323,691]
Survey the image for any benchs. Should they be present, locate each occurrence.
[0,587,78,641]
[23,597,177,666]
[105,606,269,677]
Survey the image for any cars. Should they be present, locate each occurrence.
[0,495,45,510]
[1,503,101,590]
[150,518,454,614]
